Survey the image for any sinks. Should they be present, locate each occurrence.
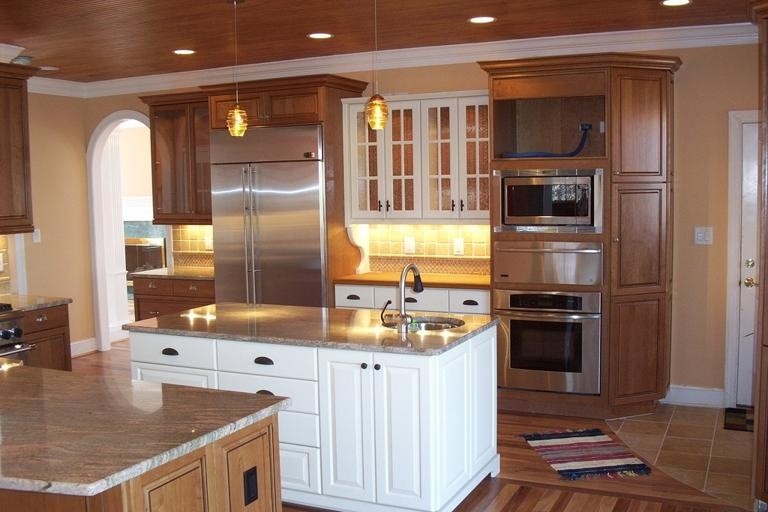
[381,315,466,332]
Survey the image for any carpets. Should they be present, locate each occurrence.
[721,405,755,433]
[519,428,652,485]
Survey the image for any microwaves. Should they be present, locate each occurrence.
[501,178,593,226]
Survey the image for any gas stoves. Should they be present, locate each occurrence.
[0,302,25,347]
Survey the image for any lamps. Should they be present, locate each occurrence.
[362,1,390,134]
[228,0,250,138]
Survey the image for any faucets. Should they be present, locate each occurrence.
[397,262,424,317]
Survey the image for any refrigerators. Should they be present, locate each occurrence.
[216,301,329,343]
[208,123,328,306]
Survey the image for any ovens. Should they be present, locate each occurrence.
[492,239,602,397]
[1,343,37,366]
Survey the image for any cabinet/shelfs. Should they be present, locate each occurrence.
[605,50,682,418]
[0,363,295,510]
[213,342,322,509]
[124,244,164,282]
[14,301,74,373]
[321,326,503,512]
[339,96,423,223]
[124,330,216,385]
[132,274,218,323]
[333,277,489,314]
[0,61,45,236]
[419,89,494,226]
[137,91,212,227]
[208,73,369,130]
